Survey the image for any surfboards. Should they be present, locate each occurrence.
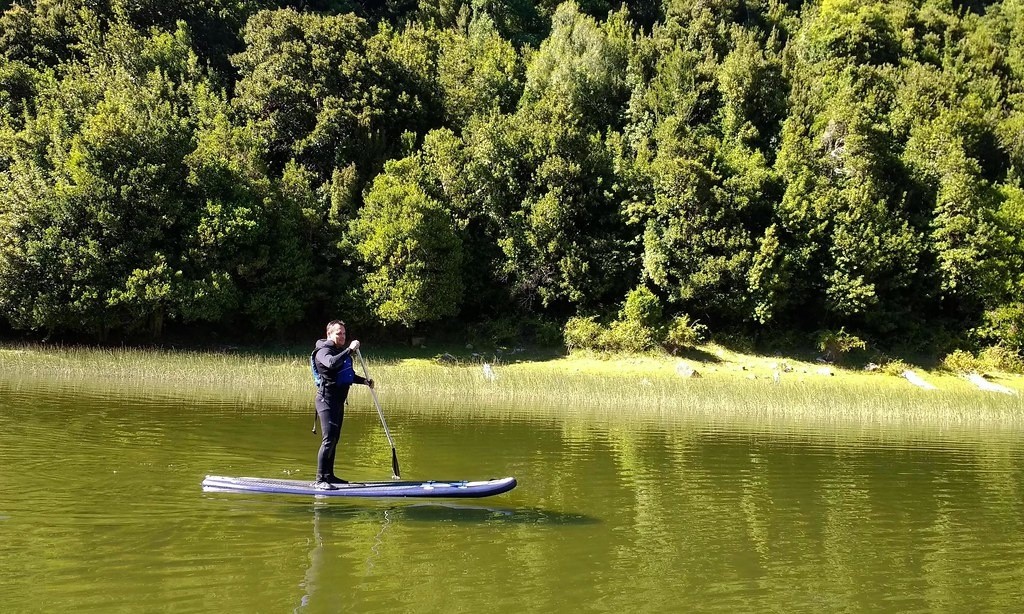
[202,474,517,500]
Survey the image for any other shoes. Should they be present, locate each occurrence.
[327,475,348,484]
[314,482,339,490]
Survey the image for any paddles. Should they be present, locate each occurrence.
[357,349,401,478]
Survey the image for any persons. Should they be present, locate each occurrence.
[310,320,374,489]
